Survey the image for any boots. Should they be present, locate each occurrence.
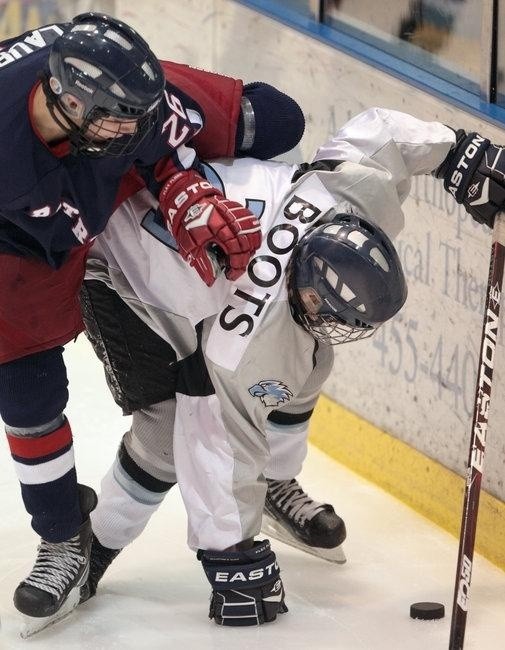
[13,481,99,613]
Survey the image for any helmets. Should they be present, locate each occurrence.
[43,11,166,159]
[285,211,408,346]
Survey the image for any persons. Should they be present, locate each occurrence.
[72,105,504,625]
[0,12,307,623]
[400,0,465,56]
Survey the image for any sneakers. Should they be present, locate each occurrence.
[264,475,345,548]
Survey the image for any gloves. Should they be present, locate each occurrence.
[160,168,262,283]
[433,123,504,230]
[197,540,286,626]
